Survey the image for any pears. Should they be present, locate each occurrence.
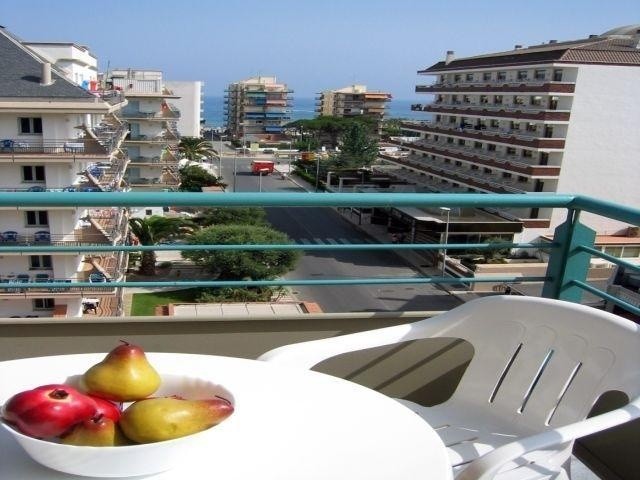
[119,394,235,444]
[78,339,161,402]
[58,413,135,445]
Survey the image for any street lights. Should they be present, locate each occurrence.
[211,128,215,142]
[440,205,450,279]
[219,135,227,177]
[258,168,269,191]
[234,147,242,191]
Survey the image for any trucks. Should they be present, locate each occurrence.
[251,160,274,175]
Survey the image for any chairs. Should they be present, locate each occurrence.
[28,186,45,192]
[63,166,101,192]
[34,231,50,242]
[0,139,14,153]
[4,272,72,291]
[2,230,17,242]
[89,272,106,283]
[256,294,640,480]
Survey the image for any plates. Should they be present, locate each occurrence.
[0,377,237,477]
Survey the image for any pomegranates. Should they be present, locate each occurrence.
[2,383,96,439]
[75,394,122,424]
